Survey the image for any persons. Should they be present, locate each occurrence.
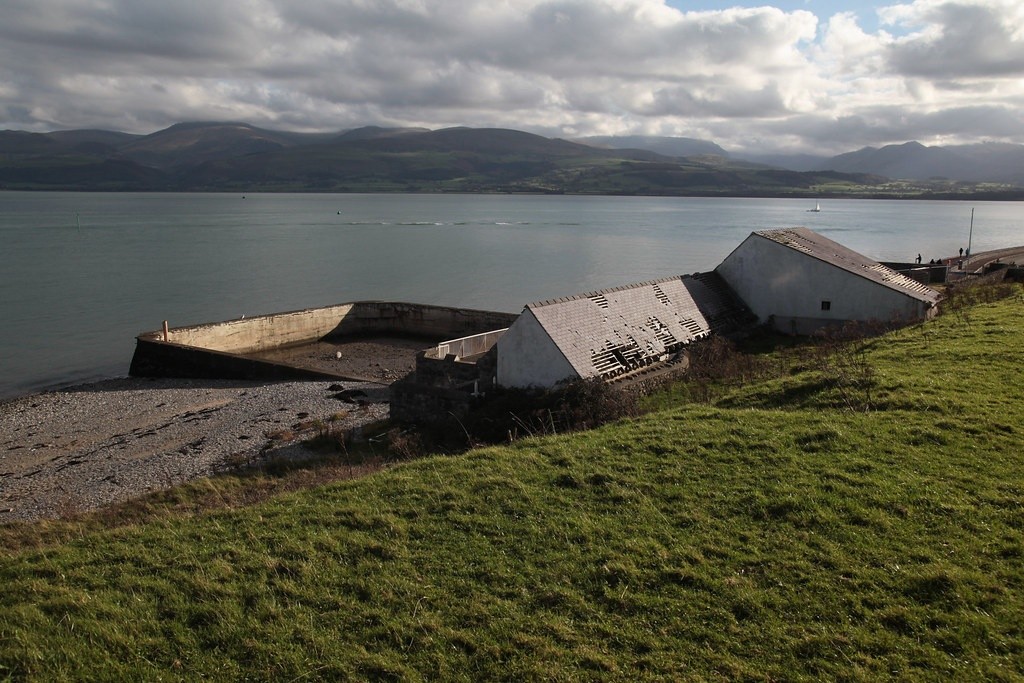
[965,248,969,257]
[930,259,935,264]
[936,259,942,264]
[959,248,963,256]
[916,254,921,263]
